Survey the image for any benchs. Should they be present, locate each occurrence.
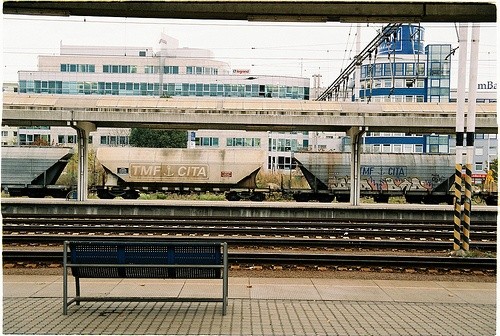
[62,238,229,315]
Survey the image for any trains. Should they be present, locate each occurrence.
[1,146,497,201]
